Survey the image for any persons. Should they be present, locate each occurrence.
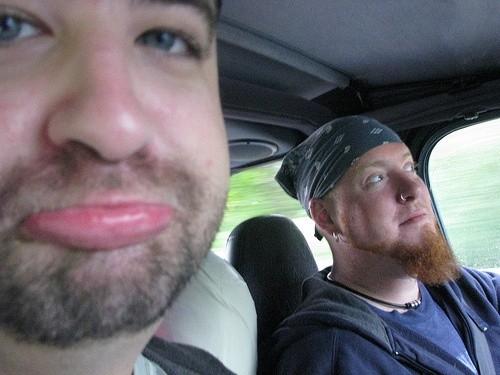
[269,115,500,375]
[0,0,259,375]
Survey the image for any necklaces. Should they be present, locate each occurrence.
[327,271,422,310]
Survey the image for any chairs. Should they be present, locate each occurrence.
[223,215,319,346]
